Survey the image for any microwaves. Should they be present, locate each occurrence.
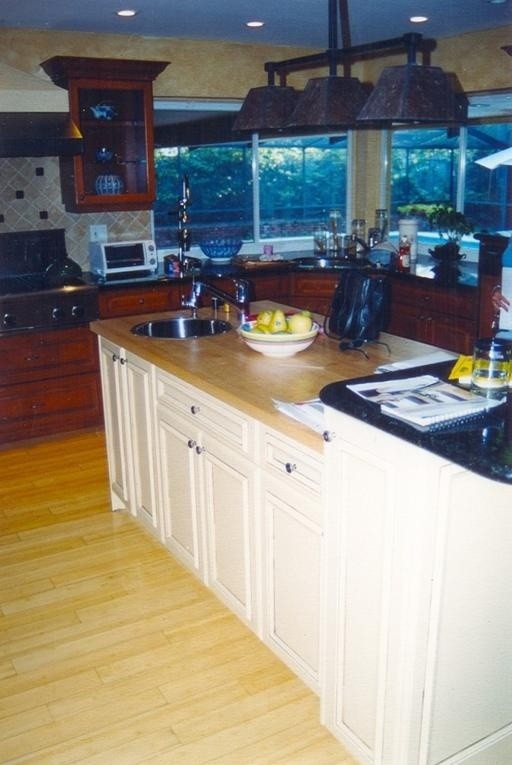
[88,238,158,277]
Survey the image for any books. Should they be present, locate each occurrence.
[347,375,507,432]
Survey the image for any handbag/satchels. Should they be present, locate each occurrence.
[324,269,392,340]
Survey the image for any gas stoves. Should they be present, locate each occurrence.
[0,259,98,338]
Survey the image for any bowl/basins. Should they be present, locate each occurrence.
[236,320,320,357]
[365,249,392,267]
[198,237,244,263]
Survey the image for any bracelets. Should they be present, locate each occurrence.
[489,284,501,293]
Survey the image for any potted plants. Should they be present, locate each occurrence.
[426,209,473,260]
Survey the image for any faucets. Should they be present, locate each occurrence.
[346,226,379,252]
[193,273,250,322]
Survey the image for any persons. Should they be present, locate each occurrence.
[489,236,512,341]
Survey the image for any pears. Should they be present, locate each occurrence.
[250,309,312,333]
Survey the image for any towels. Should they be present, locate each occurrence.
[260,254,283,262]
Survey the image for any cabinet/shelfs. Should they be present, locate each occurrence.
[180,273,290,309]
[320,405,512,765]
[385,274,479,356]
[42,55,169,212]
[258,418,322,696]
[153,362,258,633]
[97,331,159,545]
[98,283,181,319]
[0,325,104,443]
[478,241,510,338]
[291,270,391,332]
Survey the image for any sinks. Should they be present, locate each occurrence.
[130,316,233,340]
[294,254,374,273]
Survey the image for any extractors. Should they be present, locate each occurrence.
[0,112,86,158]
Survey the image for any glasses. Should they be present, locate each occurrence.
[339,340,391,359]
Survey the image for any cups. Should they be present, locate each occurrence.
[96,144,114,160]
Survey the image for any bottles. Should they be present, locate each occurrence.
[344,234,357,252]
[352,218,366,252]
[399,233,411,273]
[368,227,382,249]
[312,222,327,257]
[263,244,274,260]
[472,338,509,390]
[375,208,390,244]
[327,208,346,254]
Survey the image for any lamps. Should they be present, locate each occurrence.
[355,33,467,125]
[287,57,392,130]
[226,64,297,135]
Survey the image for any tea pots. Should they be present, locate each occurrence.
[40,249,82,285]
[89,99,121,121]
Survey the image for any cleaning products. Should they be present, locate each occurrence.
[397,236,411,270]
